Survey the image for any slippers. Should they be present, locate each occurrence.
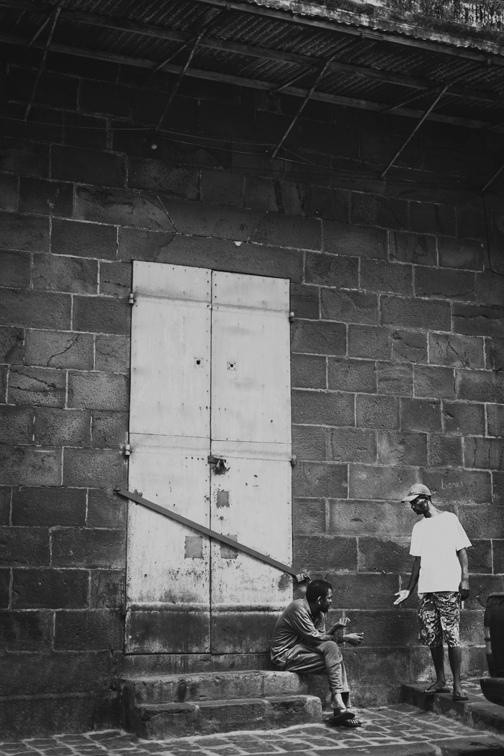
[329,710,362,727]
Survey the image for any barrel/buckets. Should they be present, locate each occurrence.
[483,591,504,680]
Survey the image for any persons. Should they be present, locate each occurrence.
[390,482,474,702]
[268,580,368,729]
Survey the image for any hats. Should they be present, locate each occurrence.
[400,483,431,502]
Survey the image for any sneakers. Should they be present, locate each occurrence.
[452,688,468,700]
[425,682,451,695]
